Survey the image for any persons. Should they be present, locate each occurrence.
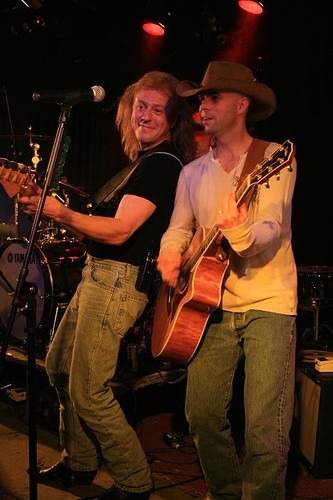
[19,71,186,500]
[157,61,298,500]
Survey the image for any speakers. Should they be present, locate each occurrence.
[293,352,333,478]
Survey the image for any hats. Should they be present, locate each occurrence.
[176,61,277,123]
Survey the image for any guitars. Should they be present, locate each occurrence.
[0,157,92,244]
[149,138,294,364]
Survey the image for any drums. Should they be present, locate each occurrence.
[36,233,85,276]
[297,264,333,310]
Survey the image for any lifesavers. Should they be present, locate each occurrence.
[0,235,88,343]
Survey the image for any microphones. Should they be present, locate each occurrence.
[30,84,106,104]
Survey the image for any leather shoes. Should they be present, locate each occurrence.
[28,461,98,486]
[75,488,149,500]
